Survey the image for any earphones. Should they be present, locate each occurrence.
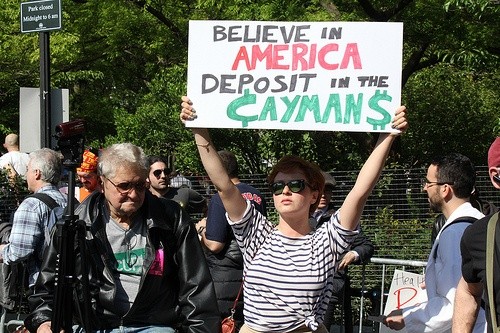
[493,175,500,182]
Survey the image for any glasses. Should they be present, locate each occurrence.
[424,177,442,185]
[270,179,312,196]
[151,168,170,177]
[103,174,151,193]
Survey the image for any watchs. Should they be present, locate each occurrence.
[197,226,206,235]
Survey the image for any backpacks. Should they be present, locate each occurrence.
[27,192,66,259]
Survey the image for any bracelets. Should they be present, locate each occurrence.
[195,138,213,150]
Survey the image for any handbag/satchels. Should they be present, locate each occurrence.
[220,317,243,333]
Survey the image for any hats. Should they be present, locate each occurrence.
[488,138,500,167]
[321,172,337,186]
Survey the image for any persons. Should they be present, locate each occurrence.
[0,133,500,333]
[180,97,409,333]
